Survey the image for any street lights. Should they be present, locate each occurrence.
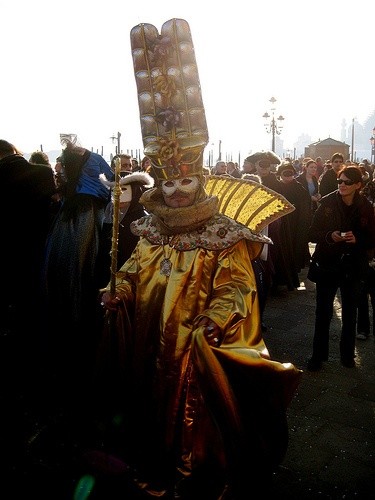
[262,96,285,154]
